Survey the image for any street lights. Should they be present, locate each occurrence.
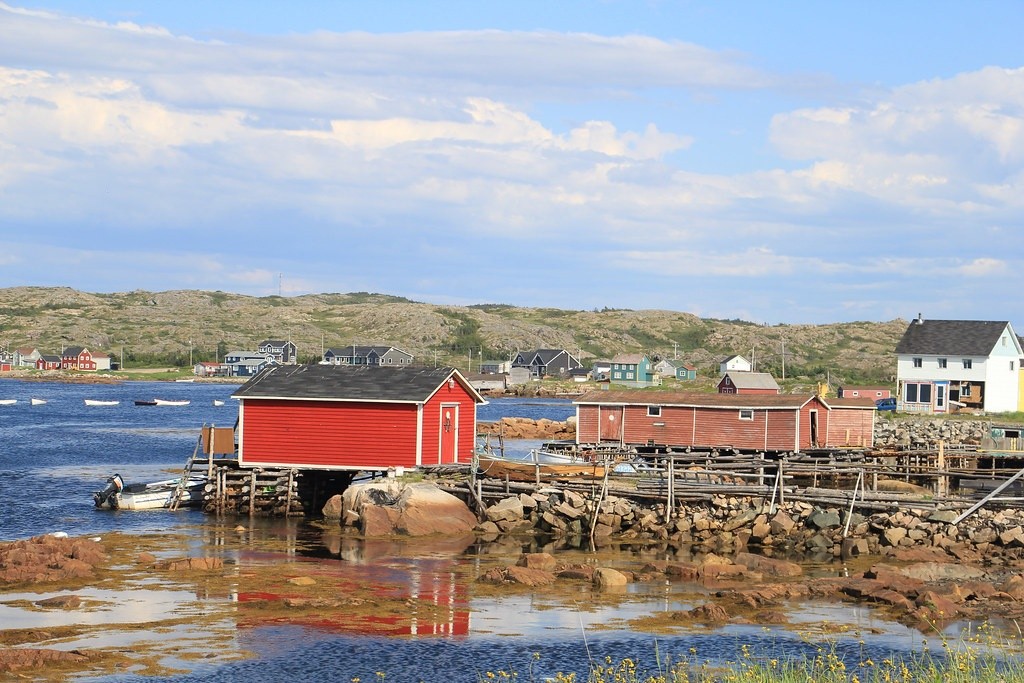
[189,340,192,367]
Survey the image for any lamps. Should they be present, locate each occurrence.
[448,377,454,388]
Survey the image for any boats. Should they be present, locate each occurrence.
[531,437,658,474]
[92,472,208,511]
[476,450,620,482]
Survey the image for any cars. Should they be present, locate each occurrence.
[875,398,896,411]
